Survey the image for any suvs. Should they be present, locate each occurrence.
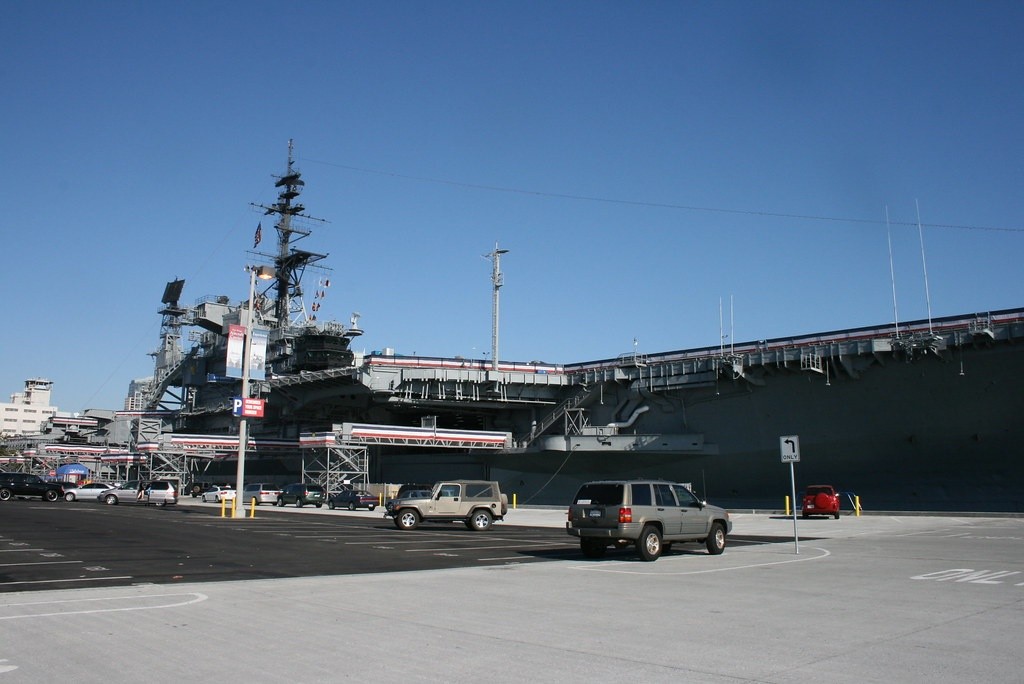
[566,478,734,563]
[0,472,66,503]
[276,483,325,508]
[386,479,509,531]
[802,484,841,520]
[191,480,212,497]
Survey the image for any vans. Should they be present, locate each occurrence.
[98,479,178,508]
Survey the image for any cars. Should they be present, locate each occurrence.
[200,484,236,503]
[384,490,431,515]
[235,483,281,505]
[327,489,380,512]
[63,481,117,503]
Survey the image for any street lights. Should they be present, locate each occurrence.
[491,247,510,372]
[230,265,277,519]
